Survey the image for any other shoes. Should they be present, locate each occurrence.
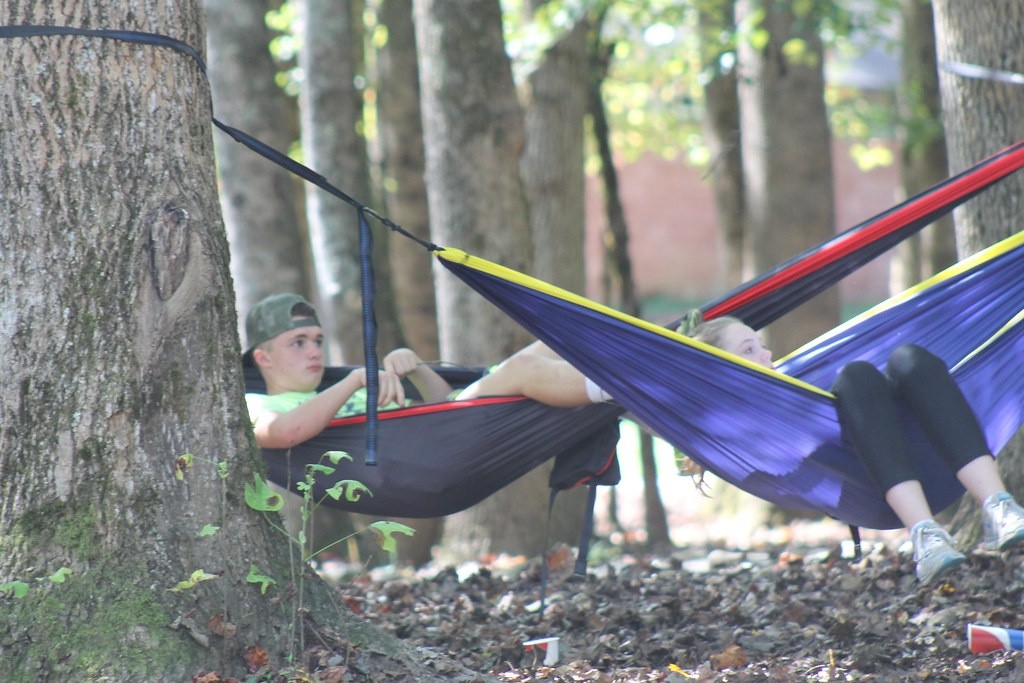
[676,306,705,335]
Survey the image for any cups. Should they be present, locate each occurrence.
[968,622,1023,654]
[523,636,560,668]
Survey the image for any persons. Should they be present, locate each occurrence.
[242,293,703,519]
[685,315,1024,587]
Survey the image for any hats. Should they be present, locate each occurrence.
[241,293,322,366]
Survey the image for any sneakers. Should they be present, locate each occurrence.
[909,519,968,587]
[981,490,1024,550]
